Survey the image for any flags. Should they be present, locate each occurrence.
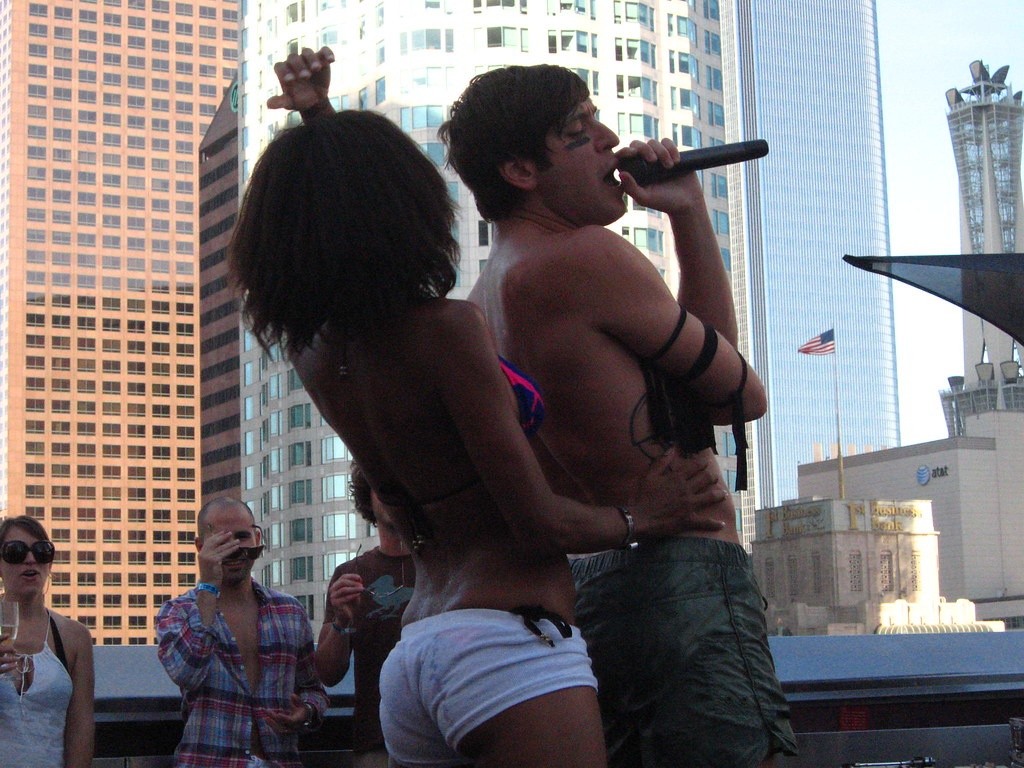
[798,329,835,356]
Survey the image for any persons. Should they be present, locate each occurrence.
[0,516,95,768]
[155,496,329,768]
[313,457,415,768]
[439,62,800,768]
[226,45,728,768]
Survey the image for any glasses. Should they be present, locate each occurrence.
[355,541,407,601]
[0,540,55,564]
[207,523,267,566]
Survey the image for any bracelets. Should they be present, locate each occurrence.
[614,502,638,552]
[196,582,221,599]
[333,623,349,632]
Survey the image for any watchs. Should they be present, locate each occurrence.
[303,703,312,727]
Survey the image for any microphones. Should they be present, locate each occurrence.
[617,139,769,188]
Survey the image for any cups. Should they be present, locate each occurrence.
[0,601,19,681]
